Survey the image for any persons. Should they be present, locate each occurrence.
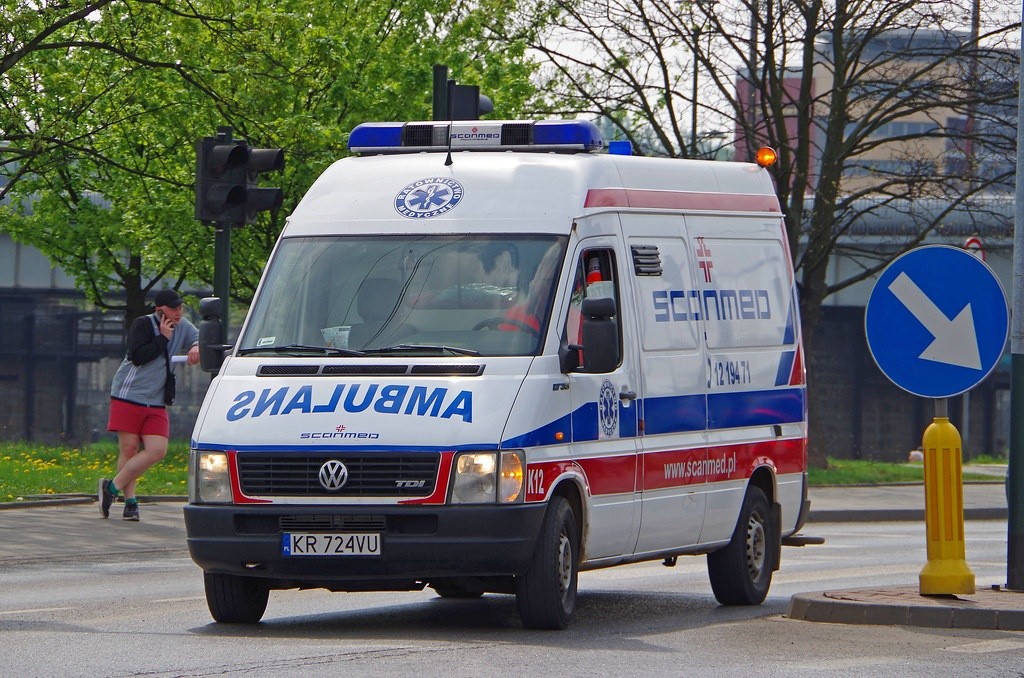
[98,288,200,520]
[500,259,584,367]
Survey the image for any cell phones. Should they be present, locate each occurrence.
[158,310,175,328]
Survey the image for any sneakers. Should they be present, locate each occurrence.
[122,502,140,521]
[99,478,118,518]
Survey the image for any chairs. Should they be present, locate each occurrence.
[347,278,417,350]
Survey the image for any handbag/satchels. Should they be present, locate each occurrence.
[164,372,177,406]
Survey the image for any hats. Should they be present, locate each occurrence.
[154,287,188,308]
